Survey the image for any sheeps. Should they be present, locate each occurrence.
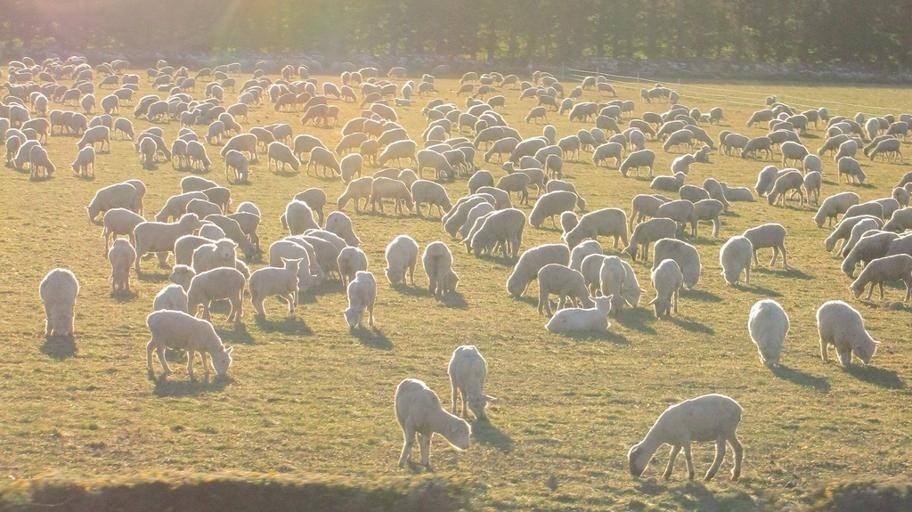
[445,343,496,426]
[709,96,910,166]
[1,52,719,175]
[38,266,80,337]
[389,377,474,470]
[85,169,908,337]
[814,297,879,374]
[143,307,234,385]
[744,295,792,371]
[627,393,745,487]
